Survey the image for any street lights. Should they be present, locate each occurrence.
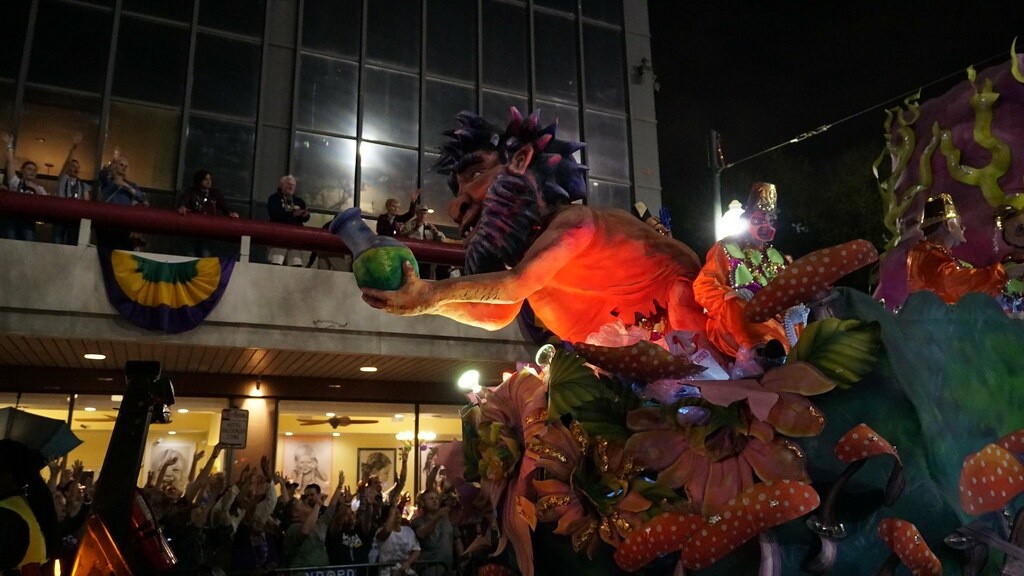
[394,430,437,508]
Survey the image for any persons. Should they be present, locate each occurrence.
[906,192,1024,304]
[175,168,239,256]
[57,133,89,245]
[98,144,150,210]
[150,449,189,499]
[423,448,446,495]
[359,452,392,491]
[288,445,327,495]
[266,176,311,266]
[358,106,702,375]
[692,182,803,372]
[48,458,479,576]
[376,188,437,240]
[0,439,54,576]
[0,132,49,242]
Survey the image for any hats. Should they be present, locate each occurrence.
[285,480,300,492]
[920,194,959,226]
[745,182,778,212]
[416,204,429,214]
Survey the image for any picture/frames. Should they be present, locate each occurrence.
[357,447,397,492]
[418,440,453,493]
[143,442,197,496]
[281,433,333,499]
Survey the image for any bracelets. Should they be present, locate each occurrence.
[7,146,13,150]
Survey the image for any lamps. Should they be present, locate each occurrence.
[719,197,750,237]
[256,375,262,390]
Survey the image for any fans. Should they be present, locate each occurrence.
[296,412,379,428]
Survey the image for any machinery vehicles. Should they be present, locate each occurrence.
[1,361,192,575]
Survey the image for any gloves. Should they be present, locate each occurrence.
[737,288,754,302]
